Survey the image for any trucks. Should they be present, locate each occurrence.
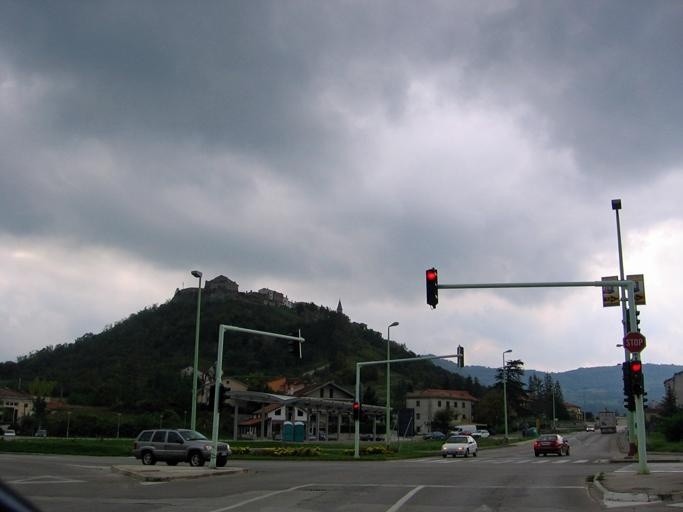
[599,411,617,434]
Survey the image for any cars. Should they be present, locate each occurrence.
[533,432,570,458]
[4,430,16,436]
[421,424,490,459]
[360,434,386,442]
[35,430,48,437]
[132,428,233,467]
[585,424,596,432]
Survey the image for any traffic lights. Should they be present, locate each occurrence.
[621,391,636,414]
[629,359,644,397]
[352,402,361,421]
[424,268,439,309]
[643,390,649,412]
[626,308,641,334]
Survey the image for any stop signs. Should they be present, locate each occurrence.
[623,331,646,352]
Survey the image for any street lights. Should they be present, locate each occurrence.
[189,269,203,434]
[502,349,513,440]
[386,321,399,451]
